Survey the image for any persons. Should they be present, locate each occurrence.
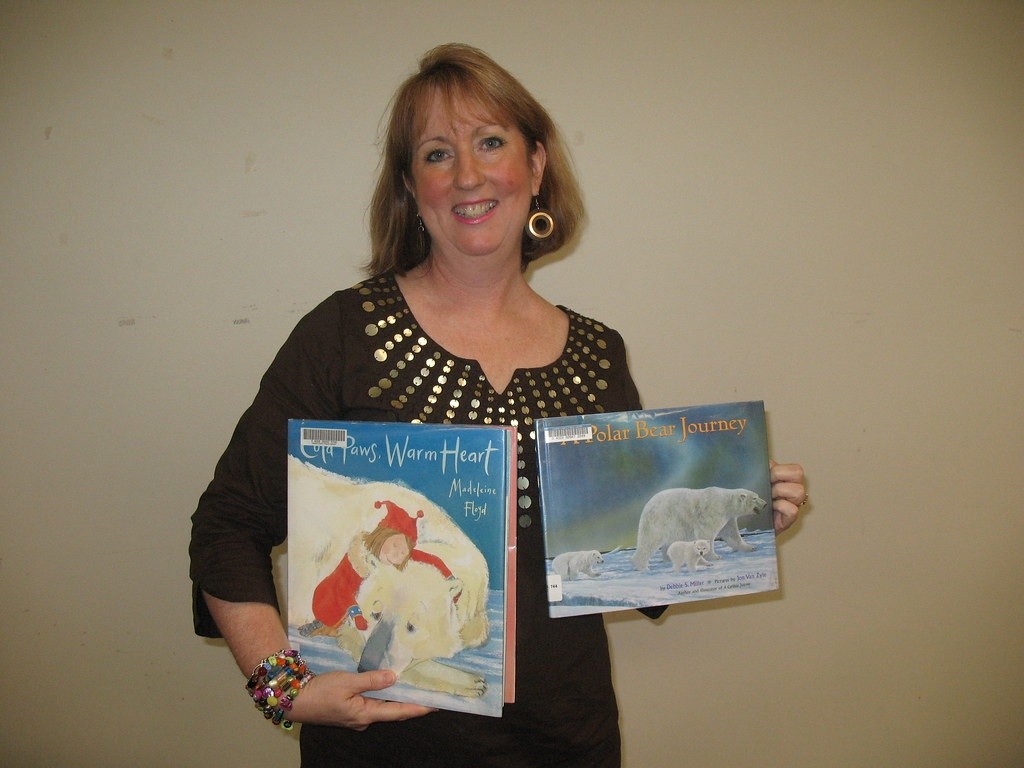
[189,42,808,768]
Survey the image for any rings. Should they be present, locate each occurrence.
[798,492,808,506]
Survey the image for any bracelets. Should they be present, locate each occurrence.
[245,650,315,730]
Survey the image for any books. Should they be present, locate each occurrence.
[287,420,518,719]
[534,399,781,618]
